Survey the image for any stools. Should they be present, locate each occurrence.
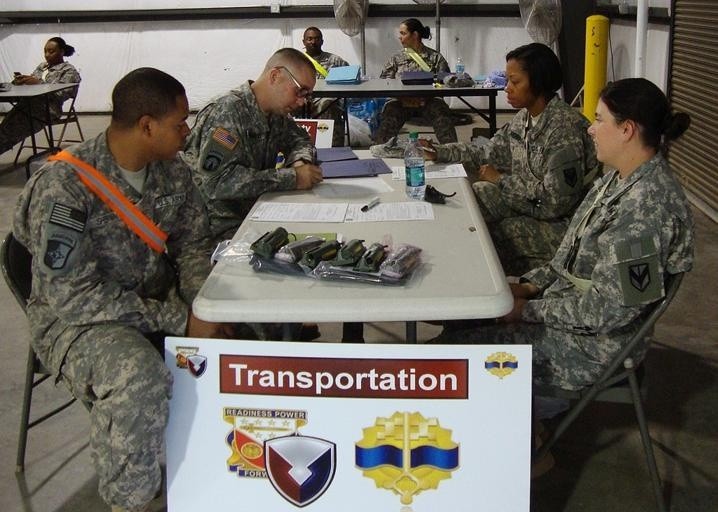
[396,113,436,138]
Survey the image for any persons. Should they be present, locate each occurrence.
[375,19,458,144]
[11,68,260,512]
[290,27,350,147]
[178,48,323,342]
[0,37,81,154]
[416,43,604,325]
[424,78,696,484]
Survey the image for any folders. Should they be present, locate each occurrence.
[316,144,392,178]
[329,65,358,85]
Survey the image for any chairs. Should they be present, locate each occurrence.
[0,223,96,480]
[25,145,64,185]
[530,267,689,511]
[14,77,85,166]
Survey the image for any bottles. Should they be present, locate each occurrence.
[404,131,427,200]
[455,52,465,79]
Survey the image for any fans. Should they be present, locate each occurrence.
[332,0,372,77]
[413,0,449,55]
[516,0,571,107]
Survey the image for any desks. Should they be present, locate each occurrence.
[299,73,509,148]
[186,147,518,345]
[0,81,81,168]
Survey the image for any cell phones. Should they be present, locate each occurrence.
[14,72,22,76]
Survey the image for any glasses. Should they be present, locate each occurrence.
[272,63,314,104]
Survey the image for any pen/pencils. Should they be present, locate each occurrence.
[422,146,436,153]
[361,196,380,211]
[311,146,317,166]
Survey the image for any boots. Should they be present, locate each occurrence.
[276,323,321,341]
